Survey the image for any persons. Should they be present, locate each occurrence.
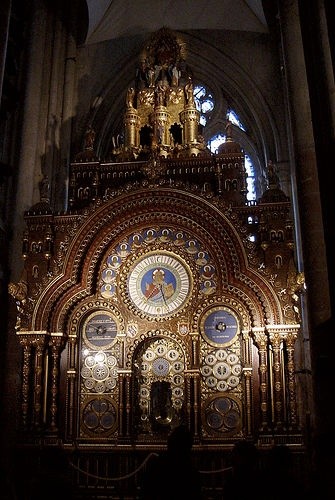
[1,429,334,500]
